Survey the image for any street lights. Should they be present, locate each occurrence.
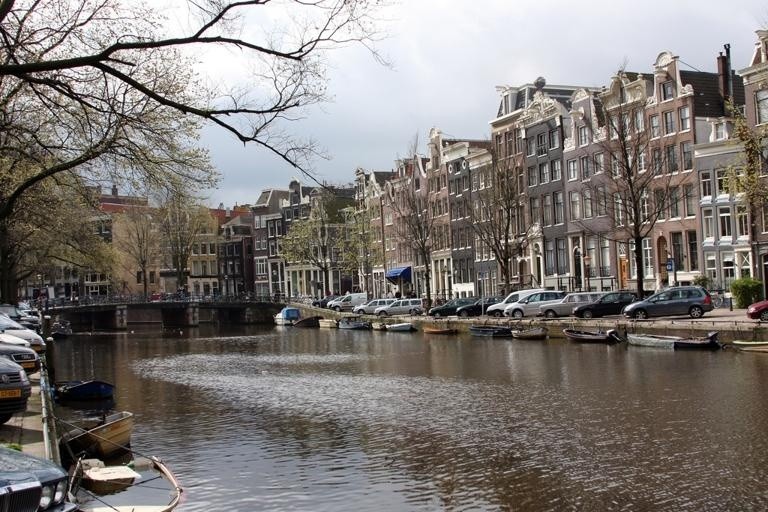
[37,274,43,299]
[272,269,279,303]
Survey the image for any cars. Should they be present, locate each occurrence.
[0,299,47,355]
[0,353,33,425]
[0,345,41,376]
[745,299,768,321]
[0,442,80,512]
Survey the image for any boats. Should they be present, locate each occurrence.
[733,338,768,353]
[293,313,324,328]
[562,326,619,343]
[316,316,552,340]
[51,322,74,340]
[51,378,116,403]
[622,330,721,350]
[271,306,301,326]
[59,408,135,459]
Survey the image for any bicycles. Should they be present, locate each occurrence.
[713,294,724,309]
